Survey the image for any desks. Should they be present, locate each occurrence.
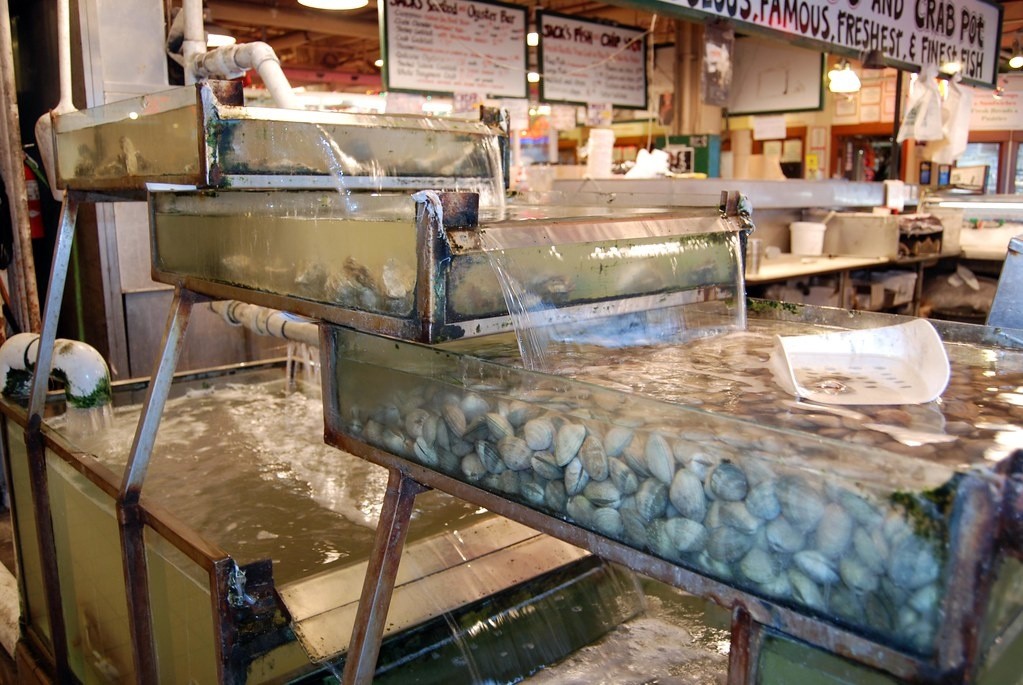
[744,247,1008,325]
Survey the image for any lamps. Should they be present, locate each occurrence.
[991,77,1009,99]
[1009,38,1023,69]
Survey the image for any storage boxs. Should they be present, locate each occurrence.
[800,210,900,260]
[850,270,917,312]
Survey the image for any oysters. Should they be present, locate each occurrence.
[215,242,723,317]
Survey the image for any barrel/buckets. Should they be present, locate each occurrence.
[789,221,827,256]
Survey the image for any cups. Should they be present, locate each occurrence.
[585,128,614,179]
